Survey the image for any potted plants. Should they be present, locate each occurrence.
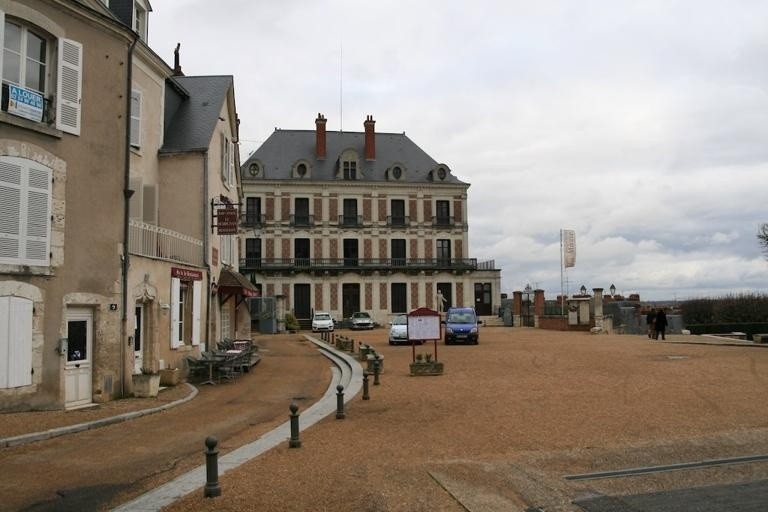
[129,366,162,399]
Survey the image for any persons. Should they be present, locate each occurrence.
[655,309,668,340]
[646,308,657,339]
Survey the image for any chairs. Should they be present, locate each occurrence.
[184,336,260,389]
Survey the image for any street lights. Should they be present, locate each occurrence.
[523,283,532,330]
[608,284,616,302]
[578,284,586,296]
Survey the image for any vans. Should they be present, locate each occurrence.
[441,307,483,346]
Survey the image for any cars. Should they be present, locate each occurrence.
[310,311,335,335]
[347,310,374,331]
[387,312,426,345]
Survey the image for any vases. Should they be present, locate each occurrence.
[157,368,180,387]
